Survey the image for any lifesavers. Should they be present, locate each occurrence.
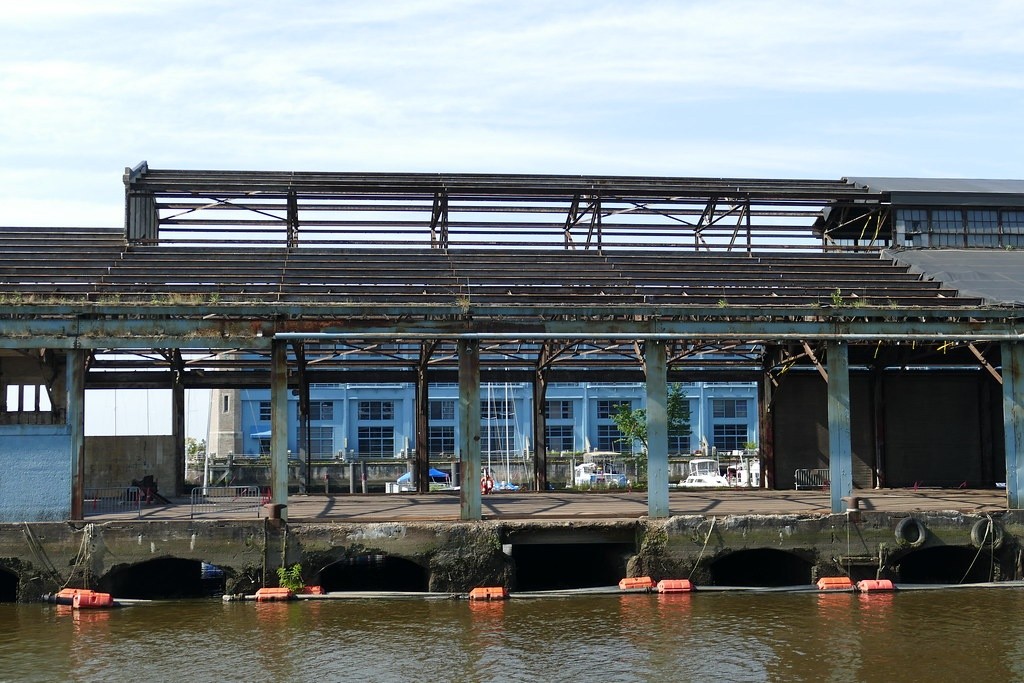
[969,518,1006,553]
[893,515,928,551]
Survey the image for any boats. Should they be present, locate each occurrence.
[676,458,730,489]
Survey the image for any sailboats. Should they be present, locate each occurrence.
[484,376,532,491]
